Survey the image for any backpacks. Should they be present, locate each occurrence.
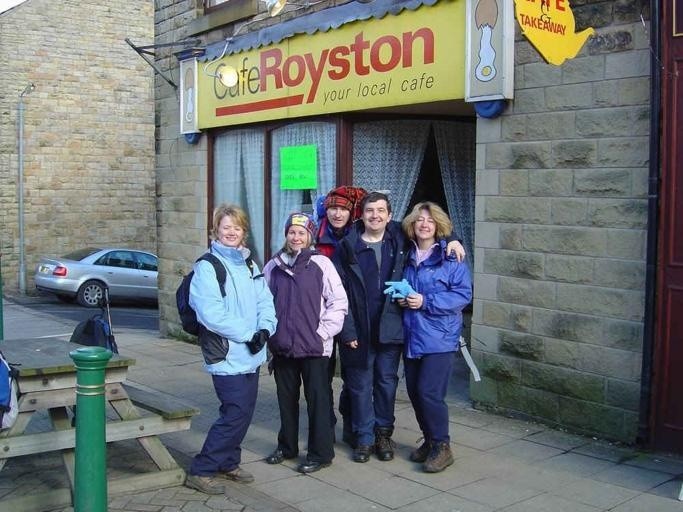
[176,252,254,335]
[69,314,118,354]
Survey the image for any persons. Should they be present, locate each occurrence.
[307,182,365,449]
[259,212,350,473]
[390,200,474,473]
[331,191,470,463]
[181,202,279,493]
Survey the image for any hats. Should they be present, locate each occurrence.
[285,213,319,240]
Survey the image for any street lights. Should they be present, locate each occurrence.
[16,80,35,299]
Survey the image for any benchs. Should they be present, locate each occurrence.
[119,382,201,421]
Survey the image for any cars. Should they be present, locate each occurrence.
[34,246,158,308]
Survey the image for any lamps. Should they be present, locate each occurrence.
[261,0,308,18]
[204,41,240,88]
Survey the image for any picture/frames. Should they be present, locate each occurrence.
[465,0,515,103]
[179,57,204,135]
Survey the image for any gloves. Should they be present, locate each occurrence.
[246,329,270,354]
[383,279,417,299]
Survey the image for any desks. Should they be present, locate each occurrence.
[0,336,201,512]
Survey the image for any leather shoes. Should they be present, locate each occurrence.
[298,459,334,473]
[268,449,286,464]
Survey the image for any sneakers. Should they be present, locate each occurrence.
[376,428,394,460]
[411,440,434,462]
[353,447,376,462]
[221,468,255,484]
[185,475,225,494]
[422,442,454,473]
